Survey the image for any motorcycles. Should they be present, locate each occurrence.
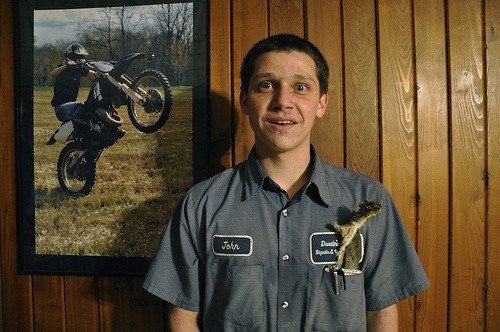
[45,53,173,196]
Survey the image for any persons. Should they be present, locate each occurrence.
[49,43,127,149]
[144,33,432,332]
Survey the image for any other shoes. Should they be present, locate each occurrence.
[104,129,126,148]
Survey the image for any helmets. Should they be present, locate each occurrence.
[63,42,89,56]
